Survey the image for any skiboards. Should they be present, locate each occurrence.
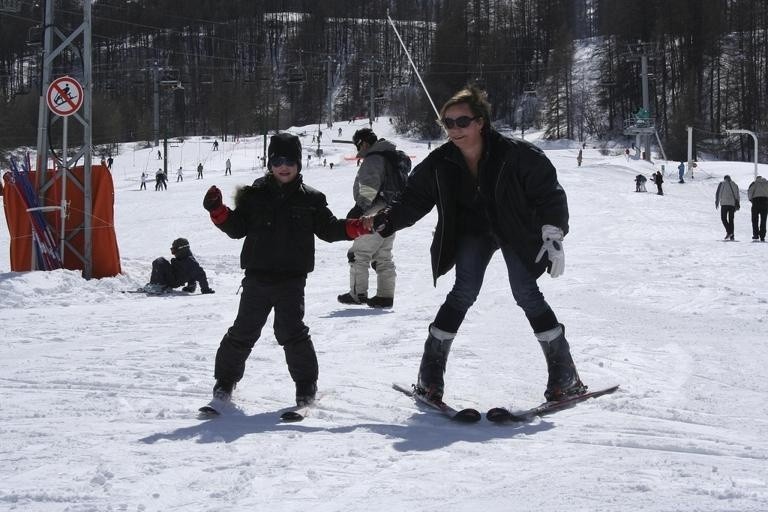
[121,290,208,296]
[199,384,325,421]
[392,382,618,422]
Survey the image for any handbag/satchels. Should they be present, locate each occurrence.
[735,199,740,209]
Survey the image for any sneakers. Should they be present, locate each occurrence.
[212,376,237,401]
[295,380,319,406]
[338,293,367,304]
[366,296,393,309]
[724,233,764,241]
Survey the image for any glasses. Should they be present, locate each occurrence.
[442,113,483,129]
[169,247,176,254]
[271,157,297,168]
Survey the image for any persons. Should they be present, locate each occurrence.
[389,118,392,124]
[213,140,219,151]
[428,141,431,150]
[133,235,217,298]
[176,167,184,182]
[678,161,685,183]
[197,163,203,179]
[298,130,361,169]
[157,150,162,160]
[52,83,70,104]
[712,173,742,245]
[650,171,664,195]
[362,83,590,407]
[577,143,586,166]
[746,174,768,243]
[100,155,113,169]
[225,159,232,176]
[635,175,647,192]
[348,116,355,124]
[192,128,371,425]
[334,125,412,310]
[140,168,168,191]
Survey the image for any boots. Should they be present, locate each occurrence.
[417,323,455,400]
[537,322,585,401]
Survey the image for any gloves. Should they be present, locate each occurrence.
[535,224,566,278]
[202,287,215,293]
[183,286,195,293]
[346,204,363,219]
[203,184,230,225]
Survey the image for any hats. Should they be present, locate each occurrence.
[173,238,190,252]
[268,133,303,159]
[352,128,376,145]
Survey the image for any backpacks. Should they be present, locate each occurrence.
[364,143,412,204]
